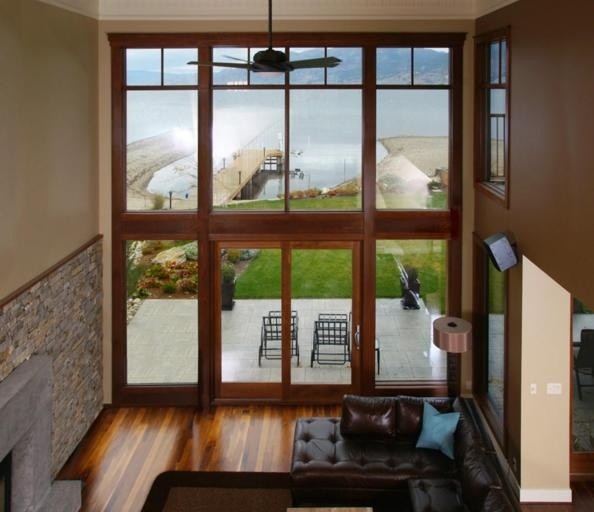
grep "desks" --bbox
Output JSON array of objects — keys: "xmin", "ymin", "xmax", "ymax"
[{"xmin": 286, "ymin": 507, "xmax": 373, "ymax": 512}]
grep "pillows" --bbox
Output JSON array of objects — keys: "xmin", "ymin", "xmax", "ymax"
[{"xmin": 415, "ymin": 401, "xmax": 461, "ymax": 460}]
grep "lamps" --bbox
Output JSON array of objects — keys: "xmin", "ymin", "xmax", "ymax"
[{"xmin": 433, "ymin": 317, "xmax": 473, "ymax": 396}]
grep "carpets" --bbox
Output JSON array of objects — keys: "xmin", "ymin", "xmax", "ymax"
[{"xmin": 140, "ymin": 471, "xmax": 293, "ymax": 512}]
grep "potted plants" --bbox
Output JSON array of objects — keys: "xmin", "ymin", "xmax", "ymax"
[{"xmin": 222, "ymin": 262, "xmax": 235, "ymax": 310}]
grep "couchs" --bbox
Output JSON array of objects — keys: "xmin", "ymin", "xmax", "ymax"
[{"xmin": 289, "ymin": 393, "xmax": 520, "ymax": 512}]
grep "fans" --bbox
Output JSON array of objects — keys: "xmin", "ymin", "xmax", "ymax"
[{"xmin": 187, "ymin": 0, "xmax": 342, "ymax": 72}]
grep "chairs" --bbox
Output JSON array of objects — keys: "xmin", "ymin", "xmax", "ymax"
[
  {"xmin": 310, "ymin": 314, "xmax": 350, "ymax": 368},
  {"xmin": 574, "ymin": 329, "xmax": 594, "ymax": 400},
  {"xmin": 258, "ymin": 310, "xmax": 299, "ymax": 367}
]
